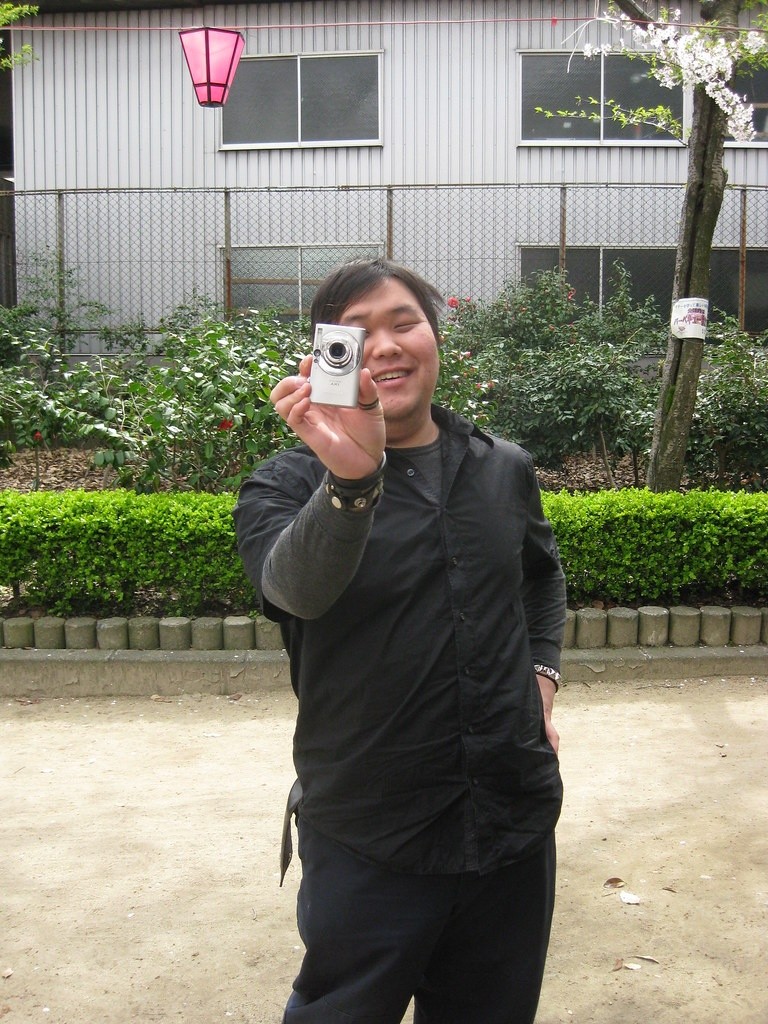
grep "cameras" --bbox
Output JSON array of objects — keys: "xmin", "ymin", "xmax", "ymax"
[{"xmin": 308, "ymin": 321, "xmax": 368, "ymax": 406}]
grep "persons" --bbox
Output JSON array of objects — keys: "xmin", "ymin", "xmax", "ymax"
[{"xmin": 227, "ymin": 257, "xmax": 570, "ymax": 1024}]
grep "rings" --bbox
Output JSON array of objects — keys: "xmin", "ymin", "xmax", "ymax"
[{"xmin": 358, "ymin": 395, "xmax": 380, "ymax": 410}]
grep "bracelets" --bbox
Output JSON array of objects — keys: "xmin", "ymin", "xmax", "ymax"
[
  {"xmin": 322, "ymin": 469, "xmax": 385, "ymax": 511},
  {"xmin": 533, "ymin": 664, "xmax": 562, "ymax": 693}
]
